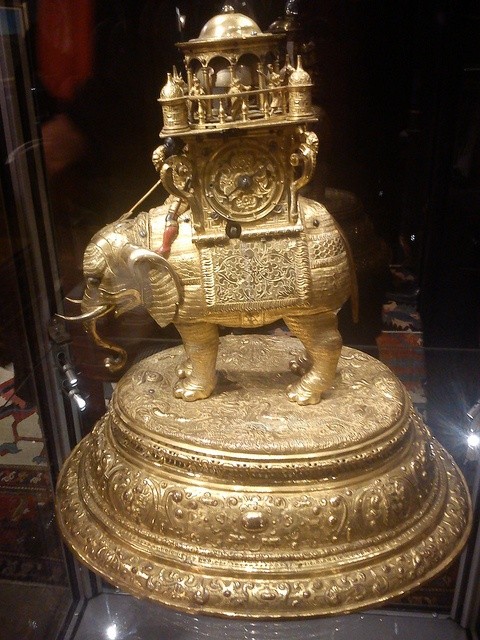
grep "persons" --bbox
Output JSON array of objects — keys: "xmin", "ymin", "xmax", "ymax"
[{"xmin": 188, "ymin": 64, "xmax": 292, "ymax": 123}]
